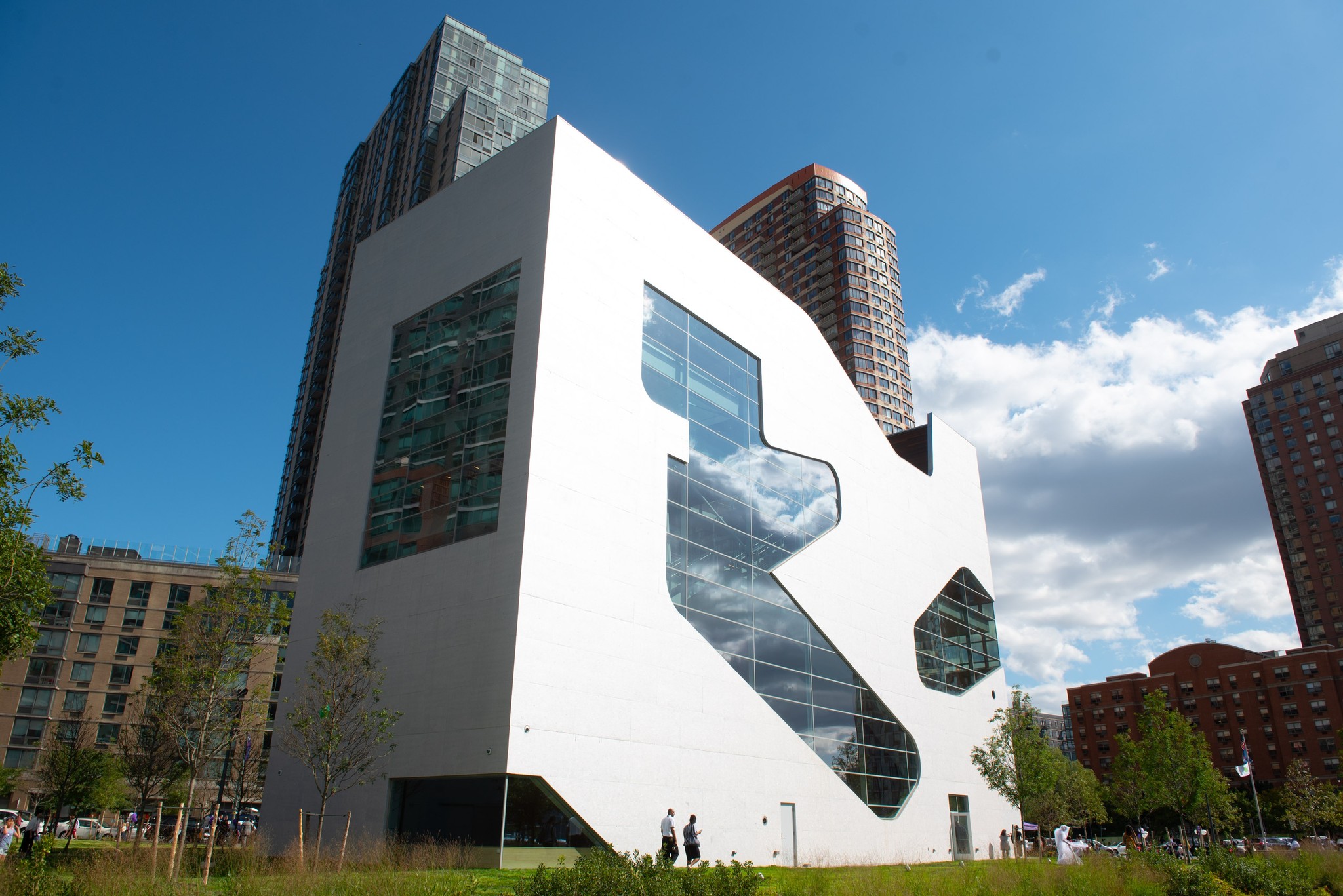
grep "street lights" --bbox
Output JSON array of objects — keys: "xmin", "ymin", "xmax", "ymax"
[{"xmin": 217, "ymin": 688, "xmax": 248, "ymax": 812}]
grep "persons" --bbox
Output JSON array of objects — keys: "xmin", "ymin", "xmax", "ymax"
[
  {"xmin": 1291, "ymin": 835, "xmax": 1337, "ymax": 851},
  {"xmin": 1230, "ymin": 836, "xmax": 1254, "ymax": 858},
  {"xmin": 121, "ymin": 820, "xmax": 129, "ymax": 842},
  {"xmin": 1010, "ymin": 825, "xmax": 1023, "ymax": 858},
  {"xmin": 213, "ymin": 814, "xmax": 256, "ymax": 848},
  {"xmin": 536, "ymin": 816, "xmax": 559, "ymax": 847},
  {"xmin": 1160, "ymin": 834, "xmax": 1194, "ymax": 860},
  {"xmin": 61, "ymin": 814, "xmax": 79, "ymax": 840},
  {"xmin": 1122, "ymin": 825, "xmax": 1136, "ymax": 861},
  {"xmin": 999, "ymin": 829, "xmax": 1010, "ymax": 859},
  {"xmin": 952, "ymin": 821, "xmax": 968, "ymax": 854},
  {"xmin": 1033, "ymin": 835, "xmax": 1046, "ymax": 850},
  {"xmin": 683, "ymin": 815, "xmax": 703, "ymax": 869},
  {"xmin": 1025, "ymin": 836, "xmax": 1028, "ymax": 850},
  {"xmin": 0, "ymin": 810, "xmax": 45, "ymax": 863},
  {"xmin": 566, "ymin": 816, "xmax": 584, "ymax": 848},
  {"xmin": 1054, "ymin": 825, "xmax": 1100, "ymax": 866},
  {"xmin": 661, "ymin": 809, "xmax": 679, "ymax": 868}
]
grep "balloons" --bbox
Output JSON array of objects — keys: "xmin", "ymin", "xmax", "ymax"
[
  {"xmin": 127, "ymin": 812, "xmax": 138, "ymax": 822},
  {"xmin": 1138, "ymin": 827, "xmax": 1148, "ymax": 839},
  {"xmin": 1194, "ymin": 829, "xmax": 1197, "ymax": 834},
  {"xmin": 1201, "ymin": 829, "xmax": 1207, "ymax": 835},
  {"xmin": 203, "ymin": 816, "xmax": 210, "ymax": 825}
]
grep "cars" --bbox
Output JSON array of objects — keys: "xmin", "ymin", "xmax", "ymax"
[
  {"xmin": 0, "ymin": 808, "xmax": 45, "ymax": 837},
  {"xmin": 1028, "ymin": 831, "xmax": 1343, "ymax": 857},
  {"xmin": 52, "ymin": 817, "xmax": 120, "ymax": 841},
  {"xmin": 126, "ymin": 822, "xmax": 150, "ymax": 840}
]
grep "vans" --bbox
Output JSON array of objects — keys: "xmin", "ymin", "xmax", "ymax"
[
  {"xmin": 143, "ymin": 814, "xmax": 204, "ymax": 844},
  {"xmin": 195, "ymin": 813, "xmax": 257, "ymax": 847}
]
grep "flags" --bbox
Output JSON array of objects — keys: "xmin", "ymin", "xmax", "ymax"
[
  {"xmin": 1240, "ymin": 732, "xmax": 1253, "ymax": 764},
  {"xmin": 1235, "ymin": 761, "xmax": 1250, "ymax": 777}
]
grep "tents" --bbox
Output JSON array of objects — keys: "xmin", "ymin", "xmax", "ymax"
[{"xmin": 1023, "ymin": 822, "xmax": 1039, "ymax": 831}]
